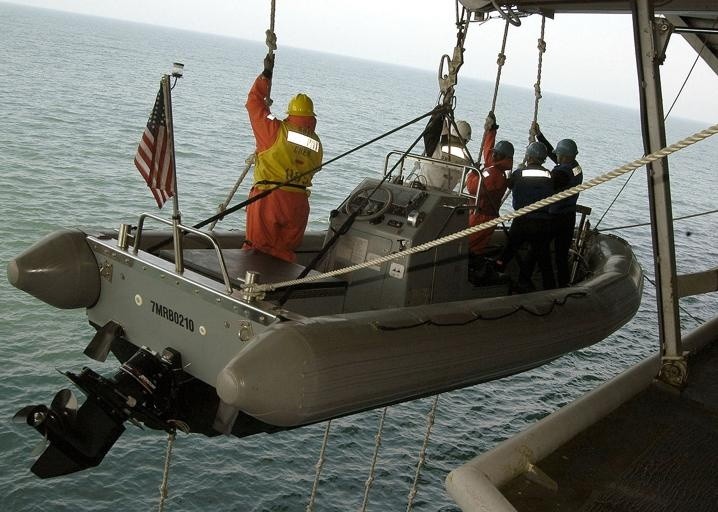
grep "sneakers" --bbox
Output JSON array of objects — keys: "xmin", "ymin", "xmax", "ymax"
[
  {"xmin": 520, "ymin": 279, "xmax": 535, "ymax": 290},
  {"xmin": 486, "ymin": 256, "xmax": 504, "ymax": 273}
]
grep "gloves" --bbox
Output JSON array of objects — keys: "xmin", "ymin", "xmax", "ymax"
[
  {"xmin": 529, "ymin": 122, "xmax": 540, "ymax": 142},
  {"xmin": 473, "ymin": 163, "xmax": 481, "ymax": 174},
  {"xmin": 488, "ymin": 111, "xmax": 499, "ymax": 129}
]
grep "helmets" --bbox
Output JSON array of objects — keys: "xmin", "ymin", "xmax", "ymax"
[
  {"xmin": 526, "ymin": 142, "xmax": 547, "ymax": 160},
  {"xmin": 552, "ymin": 139, "xmax": 578, "ymax": 156},
  {"xmin": 452, "ymin": 121, "xmax": 472, "ymax": 141},
  {"xmin": 492, "ymin": 141, "xmax": 514, "ymax": 156},
  {"xmin": 286, "ymin": 94, "xmax": 317, "ymax": 116}
]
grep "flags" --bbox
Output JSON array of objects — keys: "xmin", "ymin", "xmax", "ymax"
[{"xmin": 133, "ymin": 76, "xmax": 175, "ymax": 209}]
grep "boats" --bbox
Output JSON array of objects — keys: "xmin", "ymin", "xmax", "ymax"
[{"xmin": 7, "ymin": 148, "xmax": 642, "ymax": 480}]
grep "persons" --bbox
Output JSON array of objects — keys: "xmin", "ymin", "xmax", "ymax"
[
  {"xmin": 242, "ymin": 55, "xmax": 323, "ymax": 262},
  {"xmin": 418, "ymin": 88, "xmax": 472, "ymax": 191},
  {"xmin": 494, "ymin": 141, "xmax": 556, "ymax": 290},
  {"xmin": 534, "ymin": 123, "xmax": 583, "ymax": 289},
  {"xmin": 465, "ymin": 111, "xmax": 513, "ymax": 255}
]
